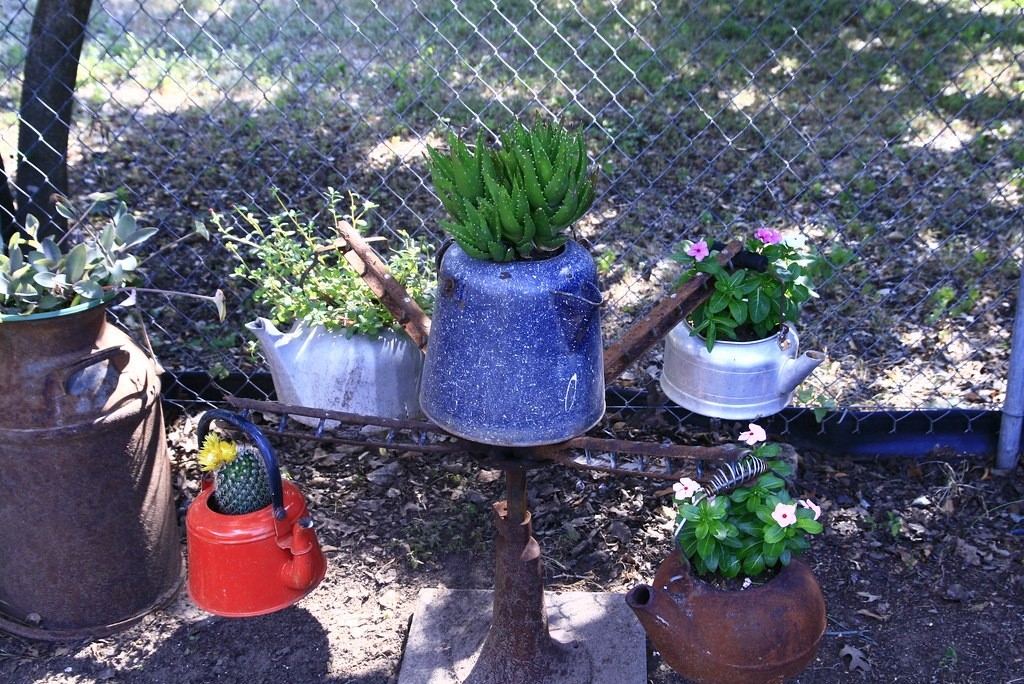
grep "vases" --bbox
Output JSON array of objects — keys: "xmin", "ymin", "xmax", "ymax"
[
  {"xmin": 187, "ymin": 477, "xmax": 327, "ymax": 616},
  {"xmin": 625, "ymin": 549, "xmax": 827, "ymax": 684},
  {"xmin": 658, "ymin": 317, "xmax": 826, "ymax": 423}
]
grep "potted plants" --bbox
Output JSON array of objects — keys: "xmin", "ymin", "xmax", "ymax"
[
  {"xmin": 0, "ymin": 189, "xmax": 228, "ymax": 643},
  {"xmin": 418, "ymin": 108, "xmax": 608, "ymax": 449},
  {"xmin": 207, "ymin": 185, "xmax": 437, "ymax": 435}
]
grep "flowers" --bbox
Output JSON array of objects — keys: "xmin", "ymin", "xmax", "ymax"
[
  {"xmin": 668, "ymin": 229, "xmax": 819, "ymax": 353},
  {"xmin": 196, "ymin": 432, "xmax": 273, "ymax": 516},
  {"xmin": 671, "ymin": 424, "xmax": 825, "ymax": 591}
]
